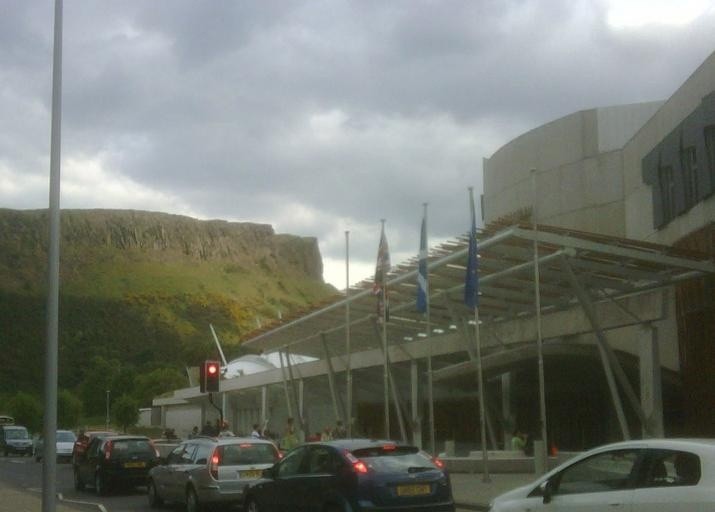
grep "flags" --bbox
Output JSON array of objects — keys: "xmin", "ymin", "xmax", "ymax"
[
  {"xmin": 416, "ymin": 218, "xmax": 426, "ymax": 310},
  {"xmin": 465, "ymin": 200, "xmax": 480, "ymax": 307},
  {"xmin": 373, "ymin": 229, "xmax": 392, "ymax": 324}
]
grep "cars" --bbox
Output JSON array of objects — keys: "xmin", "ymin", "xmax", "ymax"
[
  {"xmin": 1, "ymin": 426, "xmax": 33, "ymax": 456},
  {"xmin": 490, "ymin": 437, "xmax": 715, "ymax": 512}
]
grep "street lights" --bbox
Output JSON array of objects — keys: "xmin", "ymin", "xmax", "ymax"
[{"xmin": 105, "ymin": 390, "xmax": 111, "ymax": 431}]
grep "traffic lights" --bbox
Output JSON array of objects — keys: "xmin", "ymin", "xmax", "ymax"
[{"xmin": 205, "ymin": 361, "xmax": 220, "ymax": 393}]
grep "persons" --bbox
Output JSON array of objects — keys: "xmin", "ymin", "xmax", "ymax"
[
  {"xmin": 511, "ymin": 430, "xmax": 527, "ymax": 451},
  {"xmin": 156, "ymin": 418, "xmax": 347, "ymax": 453}
]
